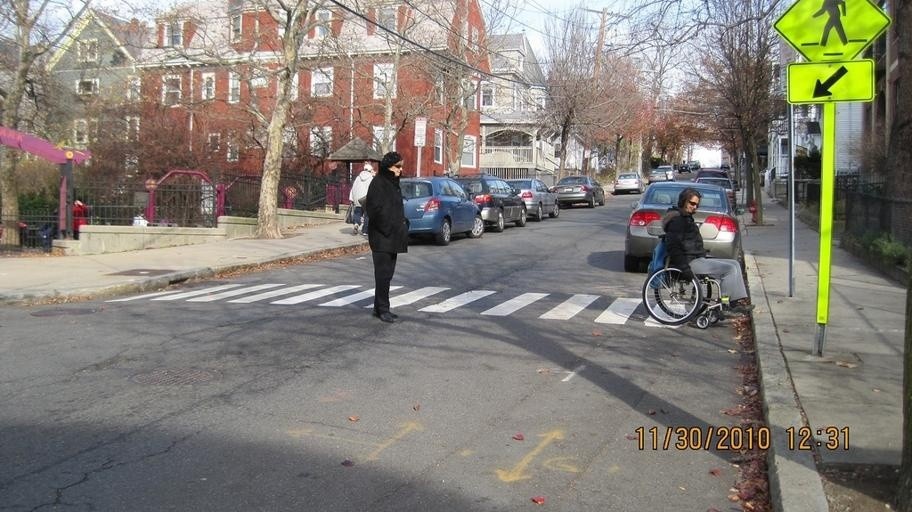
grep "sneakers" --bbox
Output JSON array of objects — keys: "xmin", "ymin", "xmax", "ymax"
[
  {"xmin": 362, "ymin": 232, "xmax": 368, "ymax": 236},
  {"xmin": 728, "ymin": 299, "xmax": 757, "ymax": 313},
  {"xmin": 353, "ymin": 225, "xmax": 359, "ymax": 235}
]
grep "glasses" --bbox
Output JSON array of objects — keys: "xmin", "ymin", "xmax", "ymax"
[
  {"xmin": 391, "ymin": 164, "xmax": 404, "ymax": 169},
  {"xmin": 688, "ymin": 200, "xmax": 700, "ymax": 209}
]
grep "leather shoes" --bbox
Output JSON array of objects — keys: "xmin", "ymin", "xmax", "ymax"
[{"xmin": 372, "ymin": 308, "xmax": 399, "ymax": 323}]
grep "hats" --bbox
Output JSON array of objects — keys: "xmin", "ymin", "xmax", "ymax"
[
  {"xmin": 380, "ymin": 151, "xmax": 402, "ymax": 168},
  {"xmin": 363, "ymin": 160, "xmax": 374, "ymax": 171}
]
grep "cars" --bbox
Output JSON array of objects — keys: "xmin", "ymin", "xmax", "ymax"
[
  {"xmin": 553, "ymin": 175, "xmax": 605, "ymax": 209},
  {"xmin": 398, "ymin": 177, "xmax": 485, "ymax": 245},
  {"xmin": 506, "ymin": 179, "xmax": 561, "ymax": 222},
  {"xmin": 612, "ymin": 159, "xmax": 741, "ymax": 272}
]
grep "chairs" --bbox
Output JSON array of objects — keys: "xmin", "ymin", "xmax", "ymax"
[
  {"xmin": 700, "ymin": 198, "xmax": 713, "ymax": 206},
  {"xmin": 656, "ymin": 194, "xmax": 672, "ymax": 204}
]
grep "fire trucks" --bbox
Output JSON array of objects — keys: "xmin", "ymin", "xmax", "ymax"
[{"xmin": 749, "ymin": 199, "xmax": 757, "ymax": 222}]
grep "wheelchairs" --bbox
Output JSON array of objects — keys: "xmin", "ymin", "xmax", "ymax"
[{"xmin": 643, "ymin": 233, "xmax": 731, "ymax": 330}]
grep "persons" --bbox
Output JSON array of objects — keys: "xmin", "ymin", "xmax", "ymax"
[
  {"xmin": 72, "ymin": 196, "xmax": 88, "ymax": 239},
  {"xmin": 365, "ymin": 150, "xmax": 410, "ymax": 323},
  {"xmin": 348, "ymin": 164, "xmax": 375, "ymax": 236},
  {"xmin": 663, "ymin": 186, "xmax": 756, "ymax": 313}
]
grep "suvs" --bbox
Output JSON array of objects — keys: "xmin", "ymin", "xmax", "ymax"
[{"xmin": 449, "ymin": 174, "xmax": 527, "ymax": 232}]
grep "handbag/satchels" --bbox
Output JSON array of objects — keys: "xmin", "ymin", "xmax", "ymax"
[{"xmin": 344, "ymin": 202, "xmax": 355, "ymax": 224}]
